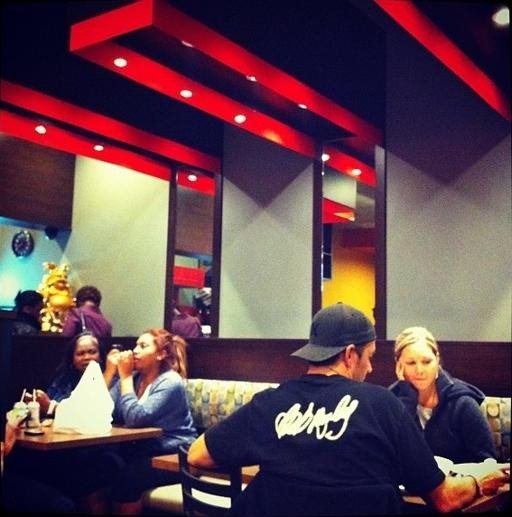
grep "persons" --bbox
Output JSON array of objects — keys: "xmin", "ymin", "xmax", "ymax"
[
  {"xmin": 63, "ymin": 286, "xmax": 112, "ymax": 337},
  {"xmin": 0, "ymin": 290, "xmax": 45, "ymax": 335},
  {"xmin": 171, "ymin": 298, "xmax": 204, "ymax": 337},
  {"xmin": 187, "ymin": 302, "xmax": 510, "ymax": 517},
  {"xmin": 387, "ymin": 327, "xmax": 497, "ymax": 463},
  {"xmin": 0, "ymin": 407, "xmax": 31, "ymax": 473},
  {"xmin": 83, "ymin": 328, "xmax": 200, "ymax": 517},
  {"xmin": 25, "ymin": 332, "xmax": 101, "ymax": 417}
]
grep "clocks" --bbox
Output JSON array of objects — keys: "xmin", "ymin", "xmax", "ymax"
[{"xmin": 13, "ymin": 230, "xmax": 34, "ymax": 257}]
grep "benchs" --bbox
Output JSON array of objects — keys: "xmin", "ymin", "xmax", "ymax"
[{"xmin": 150, "ymin": 373, "xmax": 511, "ymax": 513}]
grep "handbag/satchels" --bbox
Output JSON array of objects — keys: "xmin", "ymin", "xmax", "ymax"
[{"xmin": 52, "ymin": 359, "xmax": 114, "ymax": 435}]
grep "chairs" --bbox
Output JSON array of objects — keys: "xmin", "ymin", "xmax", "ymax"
[{"xmin": 177, "ymin": 445, "xmax": 244, "ymax": 516}]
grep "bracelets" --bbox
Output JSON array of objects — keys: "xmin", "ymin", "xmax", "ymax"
[
  {"xmin": 46, "ymin": 399, "xmax": 57, "ymax": 415},
  {"xmin": 467, "ymin": 475, "xmax": 483, "ymax": 498}
]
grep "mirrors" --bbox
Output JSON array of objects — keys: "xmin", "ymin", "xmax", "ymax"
[
  {"xmin": 165, "ymin": 162, "xmax": 223, "ymax": 340},
  {"xmin": 312, "ymin": 136, "xmax": 387, "ymax": 340}
]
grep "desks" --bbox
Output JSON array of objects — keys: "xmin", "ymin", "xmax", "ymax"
[
  {"xmin": 151, "ymin": 450, "xmax": 512, "ymax": 514},
  {"xmin": 16, "ymin": 427, "xmax": 163, "ymax": 452}
]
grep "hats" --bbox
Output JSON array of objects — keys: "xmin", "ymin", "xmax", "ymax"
[{"xmin": 290, "ymin": 301, "xmax": 376, "ymax": 362}]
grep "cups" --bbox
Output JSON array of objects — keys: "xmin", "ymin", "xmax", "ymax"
[
  {"xmin": 28, "ymin": 401, "xmax": 40, "ymax": 429},
  {"xmin": 13, "ymin": 402, "xmax": 26, "ymax": 430}
]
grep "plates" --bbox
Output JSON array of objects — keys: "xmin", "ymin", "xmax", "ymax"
[{"xmin": 449, "ymin": 461, "xmax": 505, "ymax": 479}]
